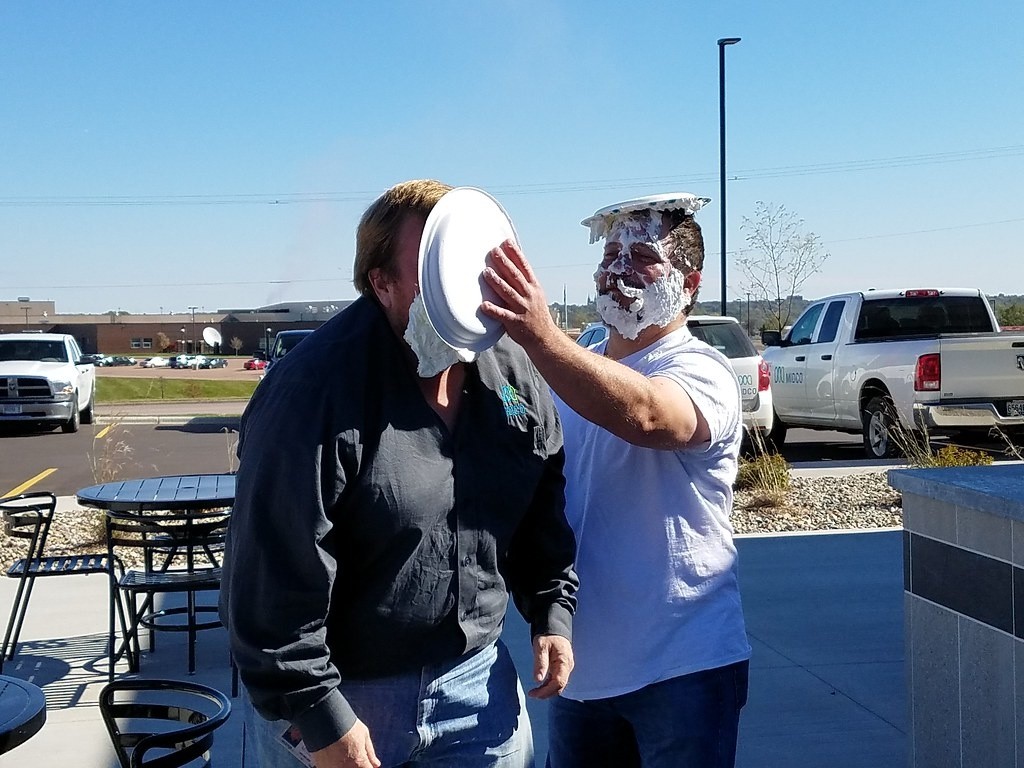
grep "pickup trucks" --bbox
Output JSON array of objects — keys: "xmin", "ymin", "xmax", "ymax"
[{"xmin": 761, "ymin": 288, "xmax": 1023, "ymax": 462}]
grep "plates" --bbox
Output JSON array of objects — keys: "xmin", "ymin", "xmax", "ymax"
[
  {"xmin": 417, "ymin": 186, "xmax": 522, "ymax": 354},
  {"xmin": 579, "ymin": 191, "xmax": 714, "ymax": 232}
]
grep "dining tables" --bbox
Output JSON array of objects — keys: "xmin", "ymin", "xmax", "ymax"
[
  {"xmin": 0, "ymin": 674, "xmax": 47, "ymax": 756},
  {"xmin": 75, "ymin": 474, "xmax": 250, "ymax": 675}
]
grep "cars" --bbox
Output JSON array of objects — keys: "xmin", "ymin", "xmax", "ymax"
[
  {"xmin": 575, "ymin": 315, "xmax": 773, "ymax": 463},
  {"xmin": 243, "ymin": 359, "xmax": 268, "ymax": 370},
  {"xmin": 265, "ymin": 329, "xmax": 317, "ymax": 375},
  {"xmin": 86, "ymin": 353, "xmax": 229, "ymax": 370},
  {"xmin": 0, "ymin": 334, "xmax": 97, "ymax": 433}
]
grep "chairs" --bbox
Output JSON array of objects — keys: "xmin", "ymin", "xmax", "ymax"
[
  {"xmin": 104, "ymin": 508, "xmax": 240, "ymax": 699},
  {"xmin": 99, "ymin": 678, "xmax": 232, "ymax": 768},
  {"xmin": 0, "ymin": 491, "xmax": 141, "ymax": 676}
]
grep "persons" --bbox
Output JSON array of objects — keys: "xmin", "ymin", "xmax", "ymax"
[
  {"xmin": 218, "ymin": 180, "xmax": 581, "ymax": 768},
  {"xmin": 478, "ymin": 205, "xmax": 751, "ymax": 767}
]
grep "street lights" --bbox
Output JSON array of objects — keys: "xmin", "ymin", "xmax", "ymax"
[
  {"xmin": 188, "ymin": 307, "xmax": 198, "ymax": 353},
  {"xmin": 266, "ymin": 327, "xmax": 271, "ymax": 360},
  {"xmin": 20, "ymin": 307, "xmax": 32, "ymax": 330},
  {"xmin": 718, "ymin": 37, "xmax": 742, "ymax": 317}
]
